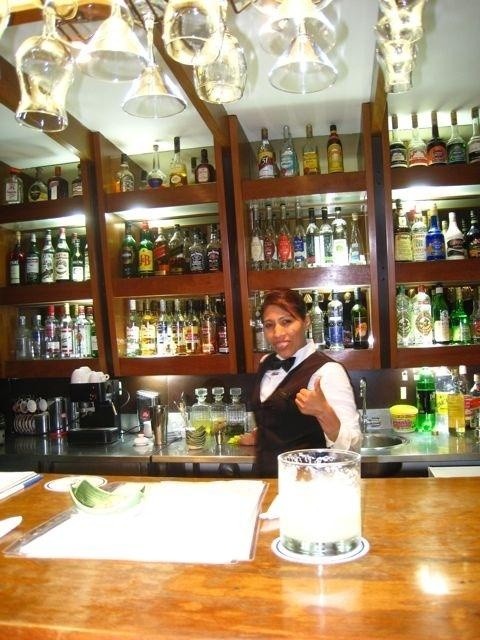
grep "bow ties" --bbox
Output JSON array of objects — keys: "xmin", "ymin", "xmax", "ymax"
[{"xmin": 264, "ymin": 355, "xmax": 296, "ymax": 373}]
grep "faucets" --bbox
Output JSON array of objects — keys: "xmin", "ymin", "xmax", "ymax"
[{"xmin": 358, "ymin": 377, "xmax": 368, "ymax": 420}]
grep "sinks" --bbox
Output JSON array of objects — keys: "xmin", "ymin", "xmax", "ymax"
[{"xmin": 360, "ymin": 433, "xmax": 403, "ymax": 450}]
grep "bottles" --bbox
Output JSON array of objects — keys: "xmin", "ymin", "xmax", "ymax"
[
  {"xmin": 280, "ymin": 125, "xmax": 299, "ymax": 177},
  {"xmin": 326, "ymin": 125, "xmax": 344, "ymax": 174},
  {"xmin": 254, "ymin": 287, "xmax": 477, "ymax": 353},
  {"xmin": 188, "ymin": 381, "xmax": 248, "ymax": 440},
  {"xmin": 388, "ymin": 108, "xmax": 480, "ymax": 169},
  {"xmin": 257, "ymin": 128, "xmax": 280, "ymax": 179},
  {"xmin": 124, "ymin": 292, "xmax": 228, "ymax": 361},
  {"xmin": 413, "ymin": 357, "xmax": 480, "ymax": 440},
  {"xmin": 189, "ymin": 148, "xmax": 215, "ymax": 184},
  {"xmin": 11, "ymin": 304, "xmax": 97, "ymax": 362},
  {"xmin": 116, "ymin": 152, "xmax": 134, "ymax": 192},
  {"xmin": 2, "ymin": 159, "xmax": 82, "ymax": 206},
  {"xmin": 141, "ymin": 145, "xmax": 167, "ymax": 189},
  {"xmin": 169, "ymin": 137, "xmax": 187, "ymax": 186},
  {"xmin": 245, "ymin": 202, "xmax": 478, "ymax": 273},
  {"xmin": 301, "ymin": 125, "xmax": 321, "ymax": 176},
  {"xmin": 9, "ymin": 225, "xmax": 90, "ymax": 285},
  {"xmin": 121, "ymin": 217, "xmax": 219, "ymax": 278}
]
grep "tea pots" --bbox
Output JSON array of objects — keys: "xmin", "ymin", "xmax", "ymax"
[{"xmin": 148, "ymin": 405, "xmax": 168, "ymax": 446}]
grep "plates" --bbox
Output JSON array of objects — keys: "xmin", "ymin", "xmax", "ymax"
[
  {"xmin": 14, "ymin": 416, "xmax": 35, "ymax": 433},
  {"xmin": 165, "ymin": 431, "xmax": 181, "ymax": 441}
]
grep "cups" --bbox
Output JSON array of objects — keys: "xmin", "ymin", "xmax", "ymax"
[
  {"xmin": 72, "ymin": 366, "xmax": 108, "ymax": 382},
  {"xmin": 136, "ymin": 390, "xmax": 162, "ymax": 434},
  {"xmin": 12, "ymin": 396, "xmax": 46, "ymax": 415},
  {"xmin": 12, "ymin": 1, "xmax": 424, "ymax": 137},
  {"xmin": 142, "ymin": 421, "xmax": 152, "ymax": 438}
]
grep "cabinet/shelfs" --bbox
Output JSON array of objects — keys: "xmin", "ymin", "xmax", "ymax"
[
  {"xmin": 105, "ymin": 181, "xmax": 238, "ymax": 373},
  {"xmin": 387, "ymin": 163, "xmax": 478, "ymax": 366},
  {"xmin": 240, "ymin": 169, "xmax": 383, "ymax": 369},
  {"xmin": 0, "ymin": 458, "xmax": 157, "ymax": 477},
  {"xmin": 2, "ymin": 196, "xmax": 106, "ymax": 374}
]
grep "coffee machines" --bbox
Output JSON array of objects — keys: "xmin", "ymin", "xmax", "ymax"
[{"xmin": 68, "ymin": 379, "xmax": 124, "ymax": 446}]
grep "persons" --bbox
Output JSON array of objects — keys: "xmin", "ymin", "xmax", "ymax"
[{"xmin": 238, "ymin": 289, "xmax": 361, "ymax": 479}]
